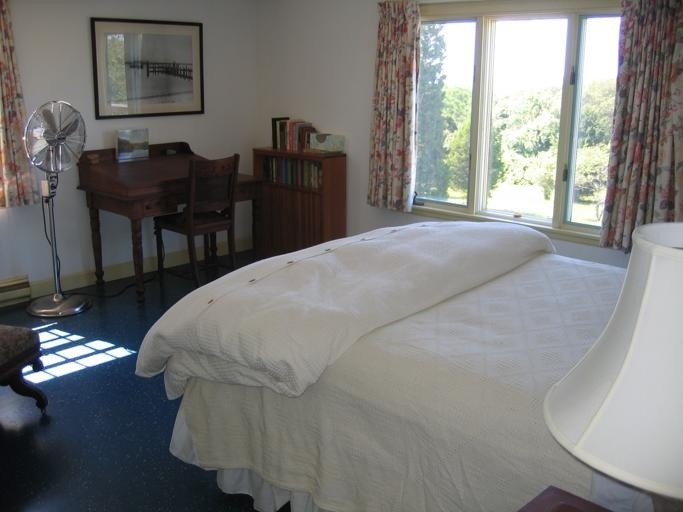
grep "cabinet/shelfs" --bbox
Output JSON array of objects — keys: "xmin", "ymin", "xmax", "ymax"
[{"xmin": 251, "ymin": 147, "xmax": 349, "ymax": 259}]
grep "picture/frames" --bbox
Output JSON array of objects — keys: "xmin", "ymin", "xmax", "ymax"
[{"xmin": 89, "ymin": 15, "xmax": 206, "ymax": 120}]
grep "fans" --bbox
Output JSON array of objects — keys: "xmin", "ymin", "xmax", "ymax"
[{"xmin": 21, "ymin": 99, "xmax": 95, "ymax": 319}]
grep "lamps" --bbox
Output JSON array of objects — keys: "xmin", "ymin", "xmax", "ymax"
[{"xmin": 544, "ymin": 221, "xmax": 683, "ymax": 511}]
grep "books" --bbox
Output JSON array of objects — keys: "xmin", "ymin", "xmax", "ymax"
[
  {"xmin": 272, "ymin": 157, "xmax": 320, "ymax": 188},
  {"xmin": 272, "ymin": 116, "xmax": 316, "ymax": 152}
]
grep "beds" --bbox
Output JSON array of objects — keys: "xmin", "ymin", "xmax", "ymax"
[{"xmin": 172, "ymin": 219, "xmax": 653, "ymax": 512}]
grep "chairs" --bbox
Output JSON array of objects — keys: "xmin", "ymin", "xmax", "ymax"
[{"xmin": 153, "ymin": 152, "xmax": 241, "ymax": 309}]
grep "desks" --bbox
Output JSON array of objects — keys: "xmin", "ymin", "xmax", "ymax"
[{"xmin": 74, "ymin": 144, "xmax": 257, "ymax": 304}]
